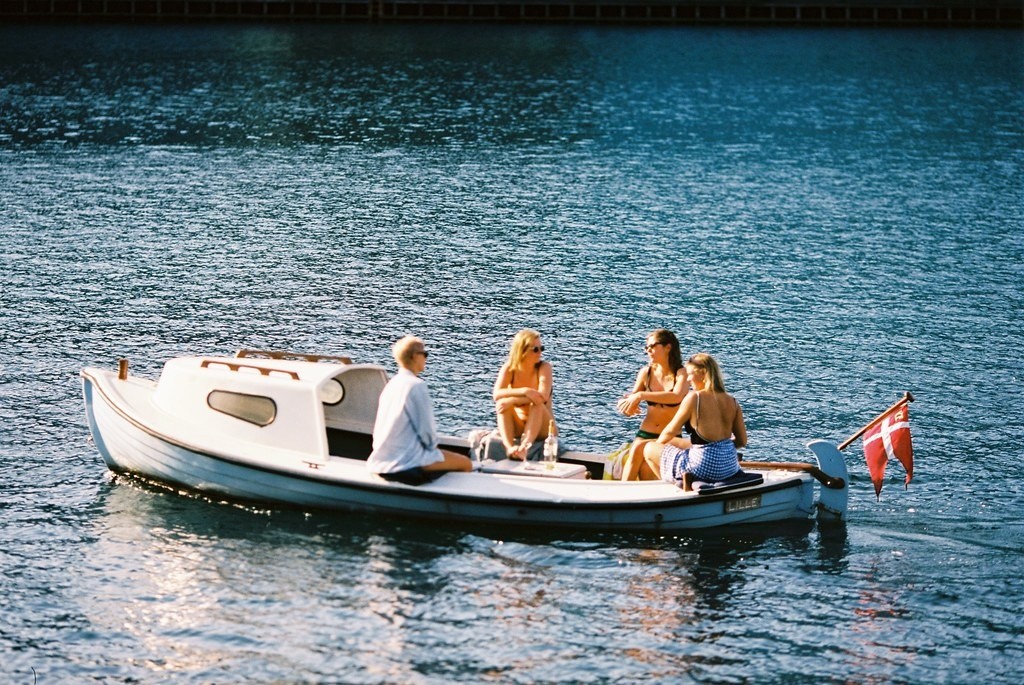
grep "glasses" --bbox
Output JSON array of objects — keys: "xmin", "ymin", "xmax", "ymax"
[
  {"xmin": 688, "ymin": 357, "xmax": 702, "ymax": 368},
  {"xmin": 527, "ymin": 345, "xmax": 545, "ymax": 353},
  {"xmin": 644, "ymin": 342, "xmax": 663, "ymax": 350},
  {"xmin": 417, "ymin": 351, "xmax": 429, "ymax": 358}
]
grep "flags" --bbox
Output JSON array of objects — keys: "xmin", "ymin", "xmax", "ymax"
[{"xmin": 864, "ymin": 403, "xmax": 914, "ymax": 502}]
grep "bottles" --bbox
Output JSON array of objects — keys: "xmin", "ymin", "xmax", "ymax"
[{"xmin": 543, "ymin": 420, "xmax": 558, "ymax": 471}]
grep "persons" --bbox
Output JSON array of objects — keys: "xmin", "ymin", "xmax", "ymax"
[
  {"xmin": 367, "ymin": 334, "xmax": 473, "ymax": 485},
  {"xmin": 617, "ymin": 328, "xmax": 689, "ymax": 482},
  {"xmin": 492, "ymin": 328, "xmax": 557, "ymax": 459},
  {"xmin": 643, "ymin": 355, "xmax": 747, "ymax": 482}
]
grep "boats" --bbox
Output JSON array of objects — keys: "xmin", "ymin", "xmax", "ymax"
[{"xmin": 81, "ymin": 345, "xmax": 850, "ymax": 532}]
